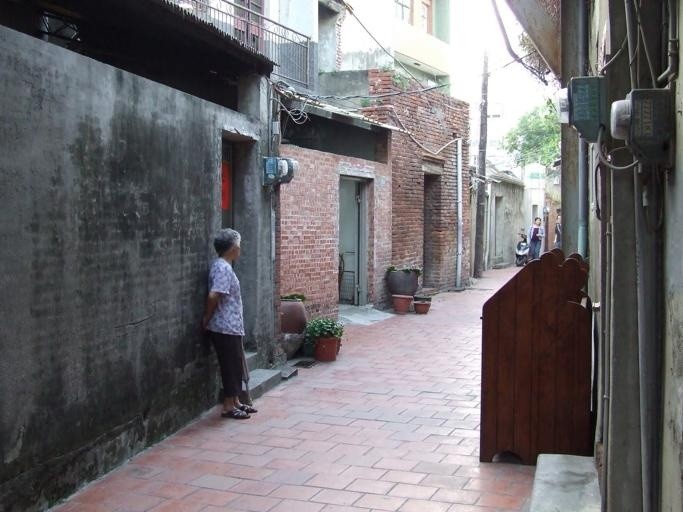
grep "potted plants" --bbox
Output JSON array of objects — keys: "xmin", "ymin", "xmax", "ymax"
[
  {"xmin": 279, "ymin": 294, "xmax": 344, "ymax": 362},
  {"xmin": 386, "ymin": 265, "xmax": 423, "ymax": 296}
]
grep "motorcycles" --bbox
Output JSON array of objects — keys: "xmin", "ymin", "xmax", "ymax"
[{"xmin": 515, "ymin": 226, "xmax": 530, "ymax": 267}]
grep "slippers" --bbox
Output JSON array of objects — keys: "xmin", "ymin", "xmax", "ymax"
[{"xmin": 221, "ymin": 404, "xmax": 257, "ymax": 418}]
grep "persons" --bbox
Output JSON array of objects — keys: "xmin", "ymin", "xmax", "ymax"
[
  {"xmin": 554, "ymin": 215, "xmax": 562, "ymax": 247},
  {"xmin": 526, "ymin": 217, "xmax": 545, "ymax": 261},
  {"xmin": 202, "ymin": 228, "xmax": 258, "ymax": 419}
]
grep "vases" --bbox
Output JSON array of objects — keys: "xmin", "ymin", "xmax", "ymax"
[{"xmin": 391, "ymin": 294, "xmax": 431, "ymax": 314}]
